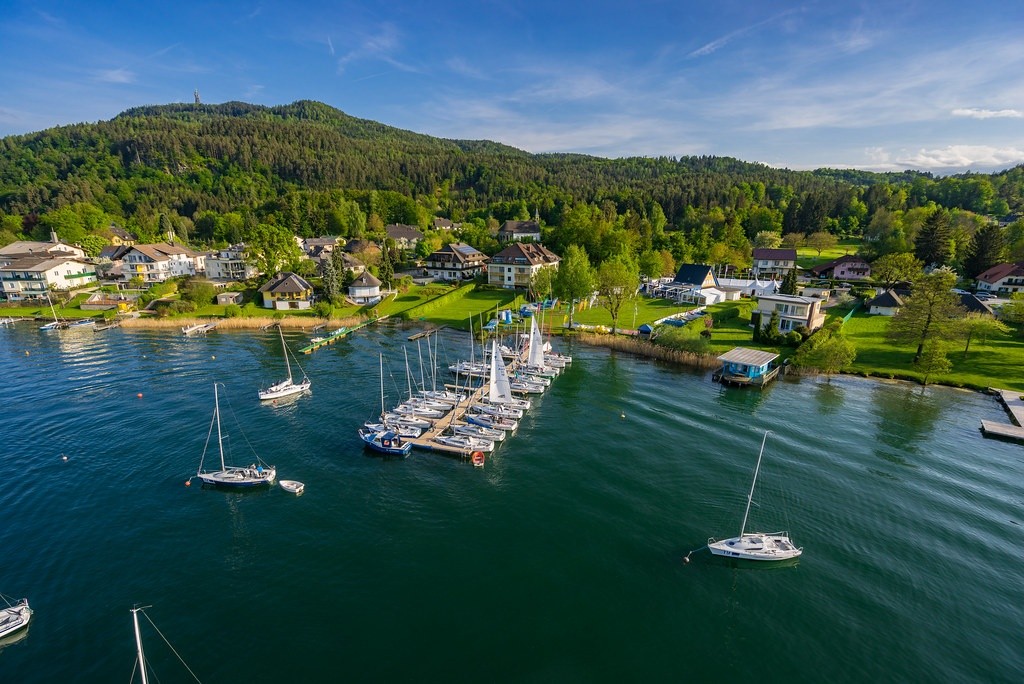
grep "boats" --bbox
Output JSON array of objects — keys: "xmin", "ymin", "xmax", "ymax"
[
  {"xmin": 68, "ymin": 318, "xmax": 96, "ymax": 329},
  {"xmin": 279, "ymin": 479, "xmax": 305, "ymax": 494},
  {"xmin": 470, "ymin": 450, "xmax": 485, "ymax": 467},
  {"xmin": 358, "ymin": 418, "xmax": 414, "ymax": 457},
  {"xmin": 311, "ymin": 336, "xmax": 324, "ymax": 345},
  {"xmin": 329, "ymin": 326, "xmax": 347, "ymax": 337}
]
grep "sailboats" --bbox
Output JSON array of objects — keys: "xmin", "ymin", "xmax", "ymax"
[
  {"xmin": 254, "ymin": 324, "xmax": 311, "ymax": 401},
  {"xmin": 195, "ymin": 380, "xmax": 277, "ymax": 489},
  {"xmin": 0, "ymin": 592, "xmax": 35, "ymax": 641},
  {"xmin": 363, "ymin": 297, "xmax": 574, "ymax": 452},
  {"xmin": 38, "ymin": 295, "xmax": 72, "ymax": 332},
  {"xmin": 706, "ymin": 428, "xmax": 805, "ymax": 562}
]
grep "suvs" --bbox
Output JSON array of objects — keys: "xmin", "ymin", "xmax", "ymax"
[
  {"xmin": 951, "ymin": 289, "xmax": 971, "ymax": 295},
  {"xmin": 975, "ymin": 292, "xmax": 997, "ymax": 299}
]
